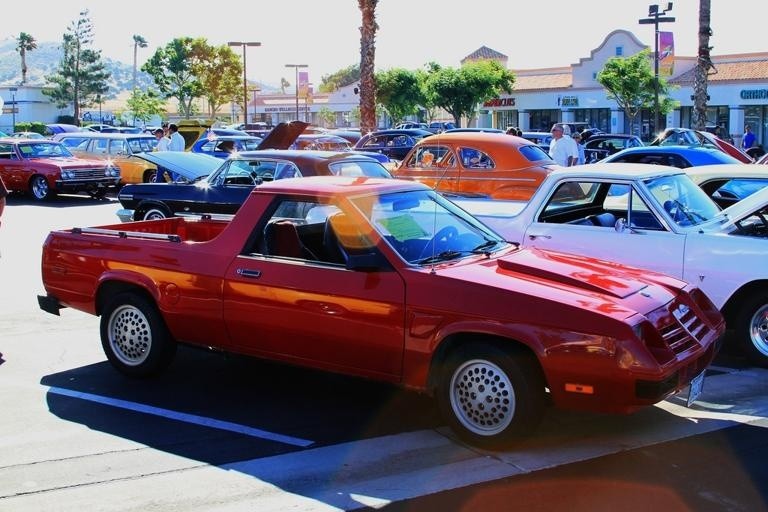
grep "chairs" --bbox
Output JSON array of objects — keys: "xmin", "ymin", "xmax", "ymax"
[
  {"xmin": 264, "ymin": 216, "xmax": 321, "ymax": 272},
  {"xmin": 320, "ymin": 211, "xmax": 373, "ymax": 266}
]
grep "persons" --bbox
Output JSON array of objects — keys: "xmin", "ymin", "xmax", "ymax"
[
  {"xmin": 741, "ymin": 124, "xmax": 757, "ymax": 148},
  {"xmin": 714, "ymin": 126, "xmax": 722, "ymax": 139},
  {"xmin": 154, "ymin": 129, "xmax": 170, "ymax": 183},
  {"xmin": 506, "ymin": 123, "xmax": 586, "ymax": 166},
  {"xmin": 0, "ymin": 177, "xmax": 9, "ymax": 259},
  {"xmin": 167, "ymin": 125, "xmax": 185, "ymax": 181},
  {"xmin": 162, "ymin": 124, "xmax": 169, "ymax": 136}
]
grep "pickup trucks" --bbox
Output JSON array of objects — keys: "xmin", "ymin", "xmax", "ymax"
[
  {"xmin": 303, "ymin": 159, "xmax": 768, "ymax": 369},
  {"xmin": 28, "ymin": 173, "xmax": 728, "ymax": 453}
]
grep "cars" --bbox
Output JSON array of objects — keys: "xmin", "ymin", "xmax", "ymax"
[
  {"xmin": 0, "ymin": 104, "xmax": 767, "ymax": 229},
  {"xmin": 0, "ymin": 138, "xmax": 123, "ymax": 202}
]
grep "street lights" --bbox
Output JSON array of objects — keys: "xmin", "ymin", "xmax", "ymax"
[
  {"xmin": 228, "ymin": 41, "xmax": 261, "ymax": 130},
  {"xmin": 284, "ymin": 64, "xmax": 311, "ymax": 120},
  {"xmin": 639, "ymin": 3, "xmax": 675, "ymax": 140}
]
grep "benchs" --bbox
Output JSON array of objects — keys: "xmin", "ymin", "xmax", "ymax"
[{"xmin": 561, "ymin": 211, "xmax": 616, "ymax": 228}]
